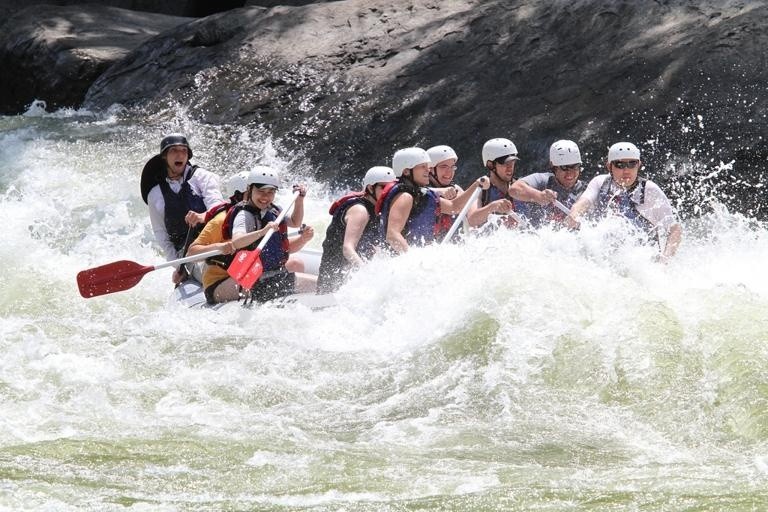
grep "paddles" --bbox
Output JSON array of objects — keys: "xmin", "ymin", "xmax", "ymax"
[
  {"xmin": 227, "ymin": 184, "xmax": 304, "ymax": 289},
  {"xmin": 76, "ymin": 224, "xmax": 307, "ymax": 298}
]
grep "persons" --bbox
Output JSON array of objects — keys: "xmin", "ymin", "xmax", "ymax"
[
  {"xmin": 566, "ymin": 142, "xmax": 682, "ymax": 263},
  {"xmin": 146, "ymin": 133, "xmax": 225, "ymax": 286},
  {"xmin": 376, "ymin": 147, "xmax": 490, "ymax": 256},
  {"xmin": 317, "ymin": 165, "xmax": 396, "ymax": 295},
  {"xmin": 234, "ymin": 167, "xmax": 319, "ymax": 302},
  {"xmin": 427, "ymin": 146, "xmax": 465, "ymax": 244},
  {"xmin": 469, "ymin": 138, "xmax": 521, "ymax": 231},
  {"xmin": 190, "ymin": 172, "xmax": 315, "ymax": 303},
  {"xmin": 510, "ymin": 139, "xmax": 590, "ymax": 230}
]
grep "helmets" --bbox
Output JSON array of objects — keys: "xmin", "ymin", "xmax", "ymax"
[
  {"xmin": 363, "ymin": 165, "xmax": 396, "ymax": 194},
  {"xmin": 426, "ymin": 145, "xmax": 458, "ymax": 168},
  {"xmin": 392, "ymin": 147, "xmax": 432, "ymax": 179},
  {"xmin": 549, "ymin": 139, "xmax": 582, "ymax": 167},
  {"xmin": 247, "ymin": 165, "xmax": 280, "ymax": 191},
  {"xmin": 481, "ymin": 137, "xmax": 518, "ymax": 168},
  {"xmin": 607, "ymin": 141, "xmax": 640, "ymax": 164},
  {"xmin": 159, "ymin": 133, "xmax": 189, "ymax": 156}
]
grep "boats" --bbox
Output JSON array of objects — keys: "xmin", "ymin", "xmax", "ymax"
[{"xmin": 163, "ymin": 227, "xmax": 326, "ymax": 320}]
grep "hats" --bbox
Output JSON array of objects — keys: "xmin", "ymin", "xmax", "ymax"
[
  {"xmin": 253, "ymin": 183, "xmax": 278, "ymax": 191},
  {"xmin": 495, "ymin": 154, "xmax": 520, "ymax": 164}
]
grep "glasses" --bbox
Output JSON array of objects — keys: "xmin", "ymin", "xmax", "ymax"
[
  {"xmin": 376, "ymin": 183, "xmax": 385, "ymax": 190},
  {"xmin": 438, "ymin": 164, "xmax": 457, "ymax": 171},
  {"xmin": 558, "ymin": 163, "xmax": 582, "ymax": 171},
  {"xmin": 611, "ymin": 160, "xmax": 638, "ymax": 169}
]
